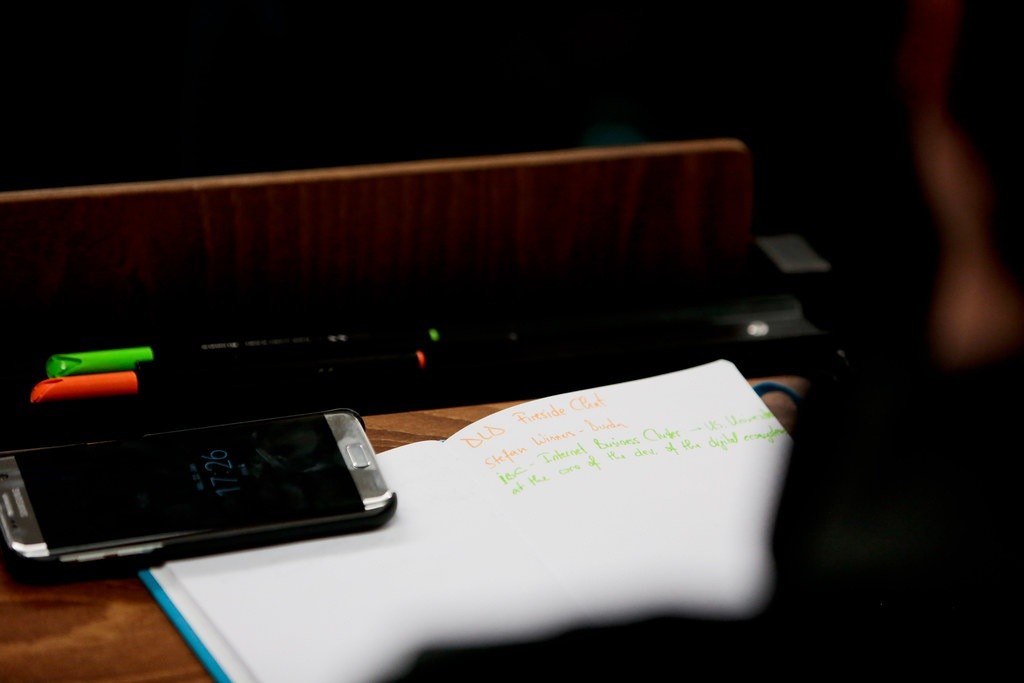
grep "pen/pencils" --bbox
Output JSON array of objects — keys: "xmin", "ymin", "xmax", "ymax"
[{"xmin": 29, "ymin": 326, "xmax": 444, "ymax": 407}]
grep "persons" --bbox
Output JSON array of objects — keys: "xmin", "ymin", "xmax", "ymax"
[{"xmin": 390, "ymin": 0, "xmax": 1023, "ymax": 683}]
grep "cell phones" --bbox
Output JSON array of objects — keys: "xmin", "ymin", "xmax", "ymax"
[{"xmin": 0, "ymin": 409, "xmax": 398, "ymax": 586}]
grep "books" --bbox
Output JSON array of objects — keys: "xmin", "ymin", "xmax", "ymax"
[{"xmin": 138, "ymin": 358, "xmax": 794, "ymax": 682}]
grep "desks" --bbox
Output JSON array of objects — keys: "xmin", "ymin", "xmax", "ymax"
[{"xmin": 0, "ymin": 401, "xmax": 521, "ymax": 682}]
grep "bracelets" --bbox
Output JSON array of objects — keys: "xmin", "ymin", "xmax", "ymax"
[{"xmin": 753, "ymin": 381, "xmax": 802, "ymax": 406}]
